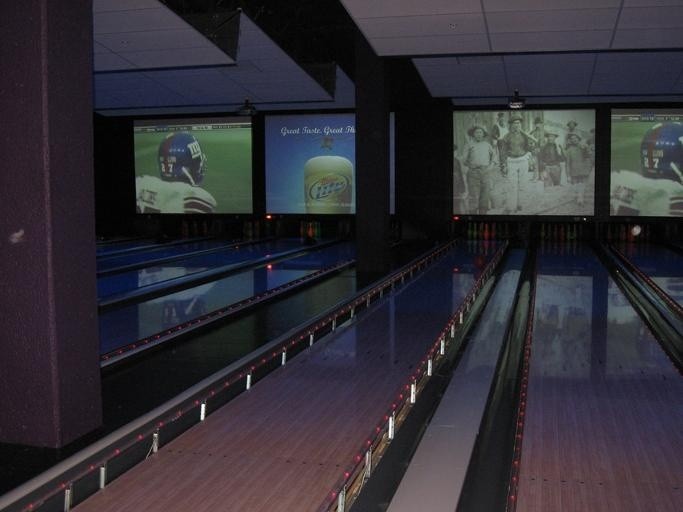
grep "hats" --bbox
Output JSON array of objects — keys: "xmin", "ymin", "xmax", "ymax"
[{"xmin": 467, "ymin": 113, "xmax": 583, "ymax": 141}]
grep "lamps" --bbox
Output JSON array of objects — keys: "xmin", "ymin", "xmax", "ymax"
[
  {"xmin": 236, "ymin": 99, "xmax": 256, "ymax": 115},
  {"xmin": 508, "ymin": 90, "xmax": 524, "ymax": 109}
]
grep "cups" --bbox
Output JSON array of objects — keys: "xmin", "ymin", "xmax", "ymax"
[{"xmin": 303, "ymin": 155, "xmax": 354, "ymax": 214}]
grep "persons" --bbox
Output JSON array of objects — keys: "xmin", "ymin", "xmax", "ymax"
[
  {"xmin": 452, "ymin": 112, "xmax": 594, "ymax": 217},
  {"xmin": 135, "ymin": 131, "xmax": 216, "ymax": 214},
  {"xmin": 609, "ymin": 121, "xmax": 682, "ymax": 216}
]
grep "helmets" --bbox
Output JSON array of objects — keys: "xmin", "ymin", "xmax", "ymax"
[
  {"xmin": 158, "ymin": 131, "xmax": 208, "ymax": 187},
  {"xmin": 640, "ymin": 120, "xmax": 683, "ymax": 181}
]
